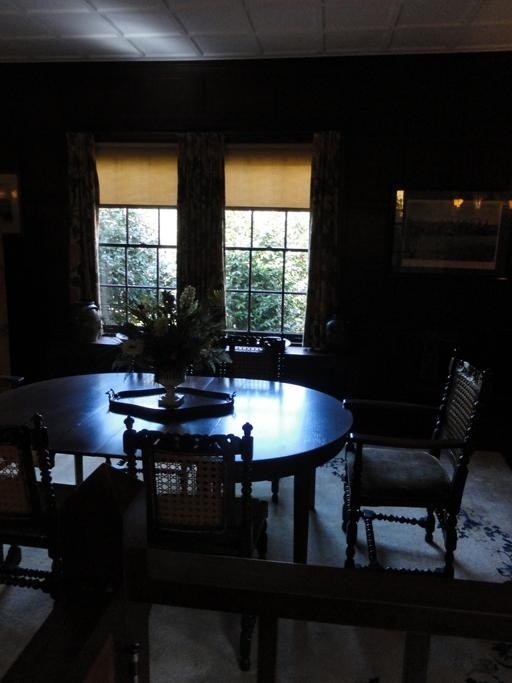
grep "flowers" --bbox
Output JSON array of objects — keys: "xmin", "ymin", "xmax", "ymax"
[{"xmin": 94, "ymin": 282, "xmax": 234, "ymax": 375}]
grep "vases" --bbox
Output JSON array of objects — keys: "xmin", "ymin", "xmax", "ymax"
[{"xmin": 154, "ymin": 367, "xmax": 186, "ymax": 408}]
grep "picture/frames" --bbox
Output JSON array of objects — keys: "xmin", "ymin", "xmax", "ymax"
[
  {"xmin": 379, "ymin": 180, "xmax": 511, "ymax": 290},
  {"xmin": 0, "ymin": 171, "xmax": 23, "ymax": 236}
]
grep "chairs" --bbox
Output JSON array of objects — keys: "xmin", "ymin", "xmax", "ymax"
[
  {"xmin": 339, "ymin": 343, "xmax": 492, "ymax": 567},
  {"xmin": 0, "ymin": 408, "xmax": 143, "ymax": 607},
  {"xmin": 122, "ymin": 413, "xmax": 269, "ymax": 560}
]
